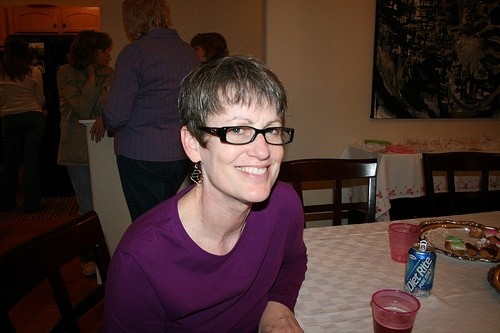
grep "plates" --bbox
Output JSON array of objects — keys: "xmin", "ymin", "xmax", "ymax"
[
  {"xmin": 418, "ymin": 220, "xmax": 500, "ymax": 263},
  {"xmin": 487, "ymin": 264, "xmax": 500, "ymax": 294}
]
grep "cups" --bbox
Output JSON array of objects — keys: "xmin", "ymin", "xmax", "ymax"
[
  {"xmin": 372, "ymin": 289, "xmax": 421, "ymax": 333},
  {"xmin": 389, "ymin": 223, "xmax": 420, "ymax": 263}
]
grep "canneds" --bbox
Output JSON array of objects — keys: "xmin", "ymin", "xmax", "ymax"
[{"xmin": 404, "ymin": 240, "xmax": 436, "ymax": 299}]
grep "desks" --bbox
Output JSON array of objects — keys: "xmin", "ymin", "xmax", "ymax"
[
  {"xmin": 294, "ymin": 212, "xmax": 500, "ymax": 333},
  {"xmin": 340, "ymin": 142, "xmax": 500, "ymax": 224}
]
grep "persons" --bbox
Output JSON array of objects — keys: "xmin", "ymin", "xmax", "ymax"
[
  {"xmin": 190, "ymin": 32, "xmax": 229, "ymax": 62},
  {"xmin": 89, "ymin": 0, "xmax": 201, "ymax": 221},
  {"xmin": 102, "ymin": 55, "xmax": 308, "ymax": 333},
  {"xmin": 57, "ymin": 29, "xmax": 114, "ymax": 216},
  {"xmin": 0, "ymin": 35, "xmax": 46, "ymax": 212}
]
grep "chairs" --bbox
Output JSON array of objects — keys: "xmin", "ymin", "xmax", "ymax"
[
  {"xmin": 276, "ymin": 158, "xmax": 378, "ymax": 228},
  {"xmin": 422, "ymin": 150, "xmax": 500, "ymax": 218},
  {"xmin": 0, "ymin": 211, "xmax": 112, "ymax": 333}
]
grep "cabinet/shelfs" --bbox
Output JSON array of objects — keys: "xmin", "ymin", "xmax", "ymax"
[{"xmin": 11, "ymin": 5, "xmax": 101, "ymax": 35}]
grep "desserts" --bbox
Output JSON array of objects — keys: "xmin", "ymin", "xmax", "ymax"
[{"xmin": 445, "ymin": 226, "xmax": 500, "ymax": 260}]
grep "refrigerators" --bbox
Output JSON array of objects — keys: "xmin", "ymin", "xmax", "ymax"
[{"xmin": 9, "ymin": 34, "xmax": 75, "ymax": 197}]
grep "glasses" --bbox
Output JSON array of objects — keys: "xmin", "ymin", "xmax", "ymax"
[{"xmin": 198, "ymin": 126, "xmax": 294, "ymax": 145}]
